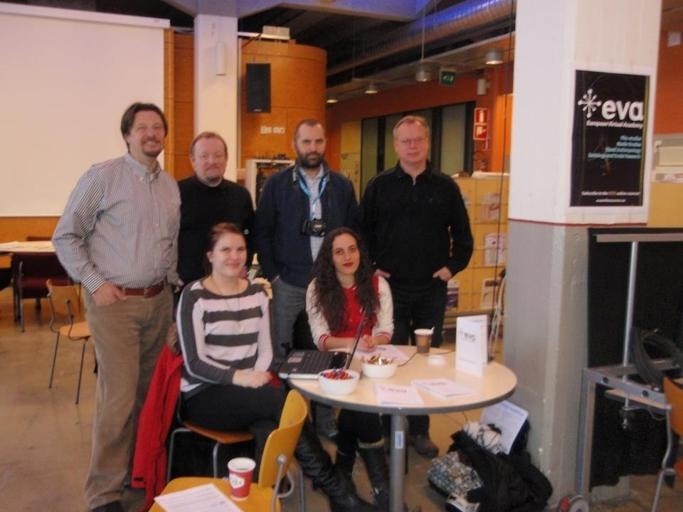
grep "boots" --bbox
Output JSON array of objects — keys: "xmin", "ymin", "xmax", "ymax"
[{"xmin": 294, "ymin": 415, "xmax": 408, "ymax": 512}]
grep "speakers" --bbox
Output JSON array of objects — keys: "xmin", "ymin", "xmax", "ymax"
[{"xmin": 245, "ymin": 63, "xmax": 271, "ymax": 113}]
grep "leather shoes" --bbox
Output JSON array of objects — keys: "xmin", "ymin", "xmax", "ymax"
[{"xmin": 410, "ymin": 434, "xmax": 438, "ymax": 458}]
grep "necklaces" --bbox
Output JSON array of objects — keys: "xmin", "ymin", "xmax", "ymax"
[{"xmin": 212, "ymin": 275, "xmax": 243, "ymax": 331}]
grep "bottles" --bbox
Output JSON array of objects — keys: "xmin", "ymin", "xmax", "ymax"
[{"xmin": 256, "ymin": 168, "xmax": 263, "ymax": 204}]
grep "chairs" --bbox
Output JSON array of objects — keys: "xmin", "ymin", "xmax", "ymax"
[
  {"xmin": 11, "ymin": 253, "xmax": 61, "ymax": 332},
  {"xmin": 25, "ymin": 234, "xmax": 75, "ymax": 287},
  {"xmin": 170, "ymin": 422, "xmax": 306, "ymax": 512},
  {"xmin": 139, "ymin": 387, "xmax": 308, "ymax": 512},
  {"xmin": 651, "ymin": 377, "xmax": 683, "ymax": 511},
  {"xmin": 44, "ymin": 279, "xmax": 93, "ymax": 404}
]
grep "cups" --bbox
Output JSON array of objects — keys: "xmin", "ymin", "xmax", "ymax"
[
  {"xmin": 414, "ymin": 328, "xmax": 433, "ymax": 353},
  {"xmin": 226, "ymin": 457, "xmax": 256, "ymax": 503}
]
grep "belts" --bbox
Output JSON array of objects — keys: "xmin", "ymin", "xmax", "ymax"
[{"xmin": 116, "ymin": 276, "xmax": 167, "ymax": 298}]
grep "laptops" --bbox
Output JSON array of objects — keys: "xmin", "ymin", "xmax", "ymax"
[{"xmin": 278, "ymin": 303, "xmax": 368, "ymax": 379}]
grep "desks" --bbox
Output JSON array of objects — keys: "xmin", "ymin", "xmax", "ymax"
[
  {"xmin": 0, "ymin": 241, "xmax": 57, "ymax": 332},
  {"xmin": 286, "ymin": 343, "xmax": 517, "ymax": 512}
]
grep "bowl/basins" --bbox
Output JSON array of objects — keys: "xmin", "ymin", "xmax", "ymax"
[
  {"xmin": 317, "ymin": 368, "xmax": 361, "ymax": 398},
  {"xmin": 361, "ymin": 355, "xmax": 397, "ymax": 379}
]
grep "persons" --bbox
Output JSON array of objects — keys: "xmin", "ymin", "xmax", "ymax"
[
  {"xmin": 178, "ymin": 221, "xmax": 379, "ymax": 512},
  {"xmin": 256, "ymin": 119, "xmax": 358, "ymax": 442},
  {"xmin": 174, "ymin": 131, "xmax": 256, "ymax": 286},
  {"xmin": 305, "ymin": 227, "xmax": 408, "ymax": 512},
  {"xmin": 358, "ymin": 113, "xmax": 475, "ymax": 459},
  {"xmin": 54, "ymin": 101, "xmax": 182, "ymax": 512}
]
